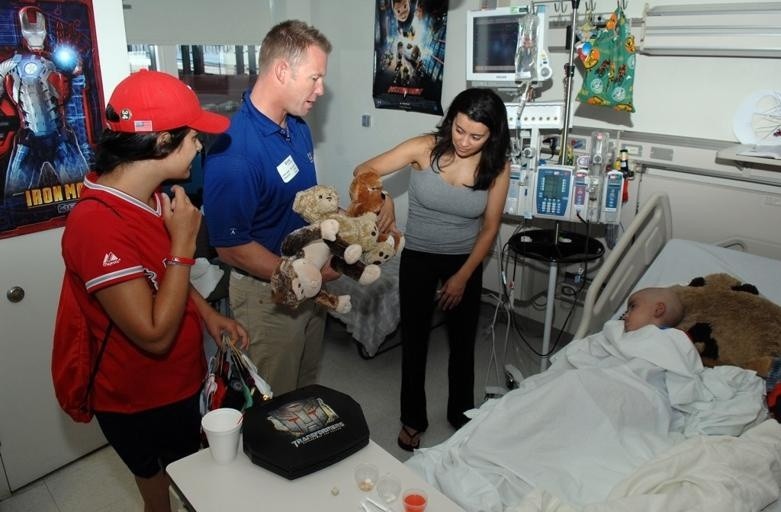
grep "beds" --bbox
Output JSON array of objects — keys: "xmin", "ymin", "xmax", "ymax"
[{"xmin": 404, "ymin": 193, "xmax": 781, "ymax": 512}]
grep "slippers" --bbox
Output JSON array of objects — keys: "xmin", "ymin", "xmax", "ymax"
[{"xmin": 397, "ymin": 424, "xmax": 421, "ymax": 452}]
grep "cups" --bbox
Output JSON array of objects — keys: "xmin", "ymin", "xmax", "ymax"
[
  {"xmin": 403, "ymin": 488, "xmax": 428, "ymax": 512},
  {"xmin": 377, "ymin": 472, "xmax": 401, "ymax": 504},
  {"xmin": 355, "ymin": 463, "xmax": 379, "ymax": 492},
  {"xmin": 201, "ymin": 407, "xmax": 243, "ymax": 463}
]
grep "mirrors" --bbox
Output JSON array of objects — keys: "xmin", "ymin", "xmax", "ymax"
[{"xmin": 127, "ymin": 44, "xmax": 261, "ymax": 104}]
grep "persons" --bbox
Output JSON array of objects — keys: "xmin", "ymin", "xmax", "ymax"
[
  {"xmin": 621, "ymin": 287, "xmax": 687, "ymax": 335},
  {"xmin": 352, "ymin": 87, "xmax": 512, "ymax": 453},
  {"xmin": 202, "ymin": 21, "xmax": 343, "ymax": 405},
  {"xmin": 390, "ymin": 1, "xmax": 420, "ymax": 39},
  {"xmin": 59, "ymin": 67, "xmax": 252, "ymax": 512}
]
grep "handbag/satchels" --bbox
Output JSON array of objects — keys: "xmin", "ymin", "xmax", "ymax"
[{"xmin": 51, "ymin": 197, "xmax": 113, "ymax": 423}]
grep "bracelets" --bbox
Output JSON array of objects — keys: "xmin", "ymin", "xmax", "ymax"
[{"xmin": 165, "ymin": 255, "xmax": 197, "ymax": 265}]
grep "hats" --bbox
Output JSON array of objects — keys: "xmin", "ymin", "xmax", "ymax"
[{"xmin": 105, "ymin": 68, "xmax": 231, "ymax": 135}]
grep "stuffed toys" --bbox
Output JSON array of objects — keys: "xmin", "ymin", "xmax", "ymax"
[
  {"xmin": 347, "ymin": 172, "xmax": 406, "ymax": 257},
  {"xmin": 292, "ymin": 184, "xmax": 396, "ymax": 268},
  {"xmin": 270, "ymin": 219, "xmax": 383, "ymax": 315}
]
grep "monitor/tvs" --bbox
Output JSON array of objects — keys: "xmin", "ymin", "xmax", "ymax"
[{"xmin": 465, "ymin": 5, "xmax": 552, "ymax": 91}]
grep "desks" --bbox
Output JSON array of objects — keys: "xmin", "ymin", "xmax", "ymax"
[{"xmin": 165, "ymin": 438, "xmax": 468, "ymax": 512}]
